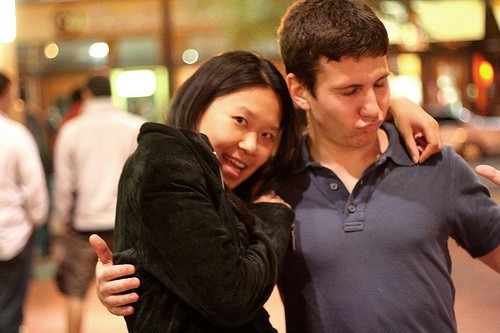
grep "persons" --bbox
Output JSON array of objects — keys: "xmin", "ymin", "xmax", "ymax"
[
  {"xmin": 0, "ymin": 74, "xmax": 153, "ymax": 333},
  {"xmin": 88, "ymin": 0, "xmax": 500, "ymax": 333},
  {"xmin": 111, "ymin": 48, "xmax": 444, "ymax": 332}
]
